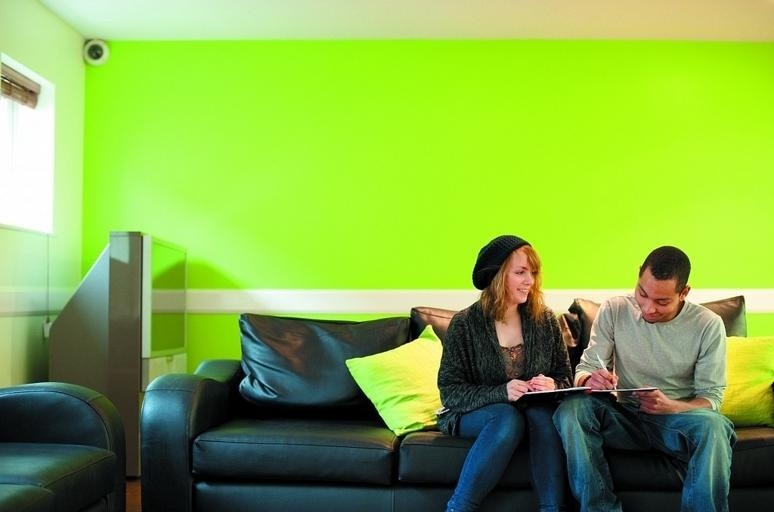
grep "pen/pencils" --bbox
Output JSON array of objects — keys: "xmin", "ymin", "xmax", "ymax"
[{"xmin": 597, "ymin": 354, "xmax": 617, "ymax": 390}]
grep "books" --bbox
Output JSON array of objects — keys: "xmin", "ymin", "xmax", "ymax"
[
  {"xmin": 592, "ymin": 387, "xmax": 658, "ymax": 394},
  {"xmin": 514, "ymin": 386, "xmax": 595, "ymax": 404}
]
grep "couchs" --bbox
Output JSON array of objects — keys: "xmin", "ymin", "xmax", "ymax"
[
  {"xmin": 0, "ymin": 383, "xmax": 126, "ymax": 512},
  {"xmin": 137, "ymin": 311, "xmax": 774, "ymax": 512}
]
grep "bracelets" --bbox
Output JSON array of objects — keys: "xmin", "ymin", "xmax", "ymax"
[{"xmin": 581, "ymin": 375, "xmax": 591, "ymax": 387}]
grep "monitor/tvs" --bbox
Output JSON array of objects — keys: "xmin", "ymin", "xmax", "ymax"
[{"xmin": 141, "ymin": 234, "xmax": 188, "ymax": 358}]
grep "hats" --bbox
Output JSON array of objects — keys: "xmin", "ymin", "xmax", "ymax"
[{"xmin": 472, "ymin": 233, "xmax": 533, "ymax": 291}]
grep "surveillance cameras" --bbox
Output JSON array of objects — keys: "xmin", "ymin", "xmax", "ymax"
[{"xmin": 83, "ymin": 39, "xmax": 109, "ymax": 65}]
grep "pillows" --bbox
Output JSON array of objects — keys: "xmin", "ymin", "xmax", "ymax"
[
  {"xmin": 410, "ymin": 308, "xmax": 583, "ymax": 381},
  {"xmin": 237, "ymin": 313, "xmax": 414, "ymax": 420},
  {"xmin": 568, "ymin": 295, "xmax": 745, "ymax": 405},
  {"xmin": 716, "ymin": 337, "xmax": 774, "ymax": 429},
  {"xmin": 346, "ymin": 326, "xmax": 451, "ymax": 437}
]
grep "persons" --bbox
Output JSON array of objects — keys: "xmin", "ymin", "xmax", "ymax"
[
  {"xmin": 434, "ymin": 234, "xmax": 575, "ymax": 512},
  {"xmin": 552, "ymin": 244, "xmax": 741, "ymax": 512}
]
